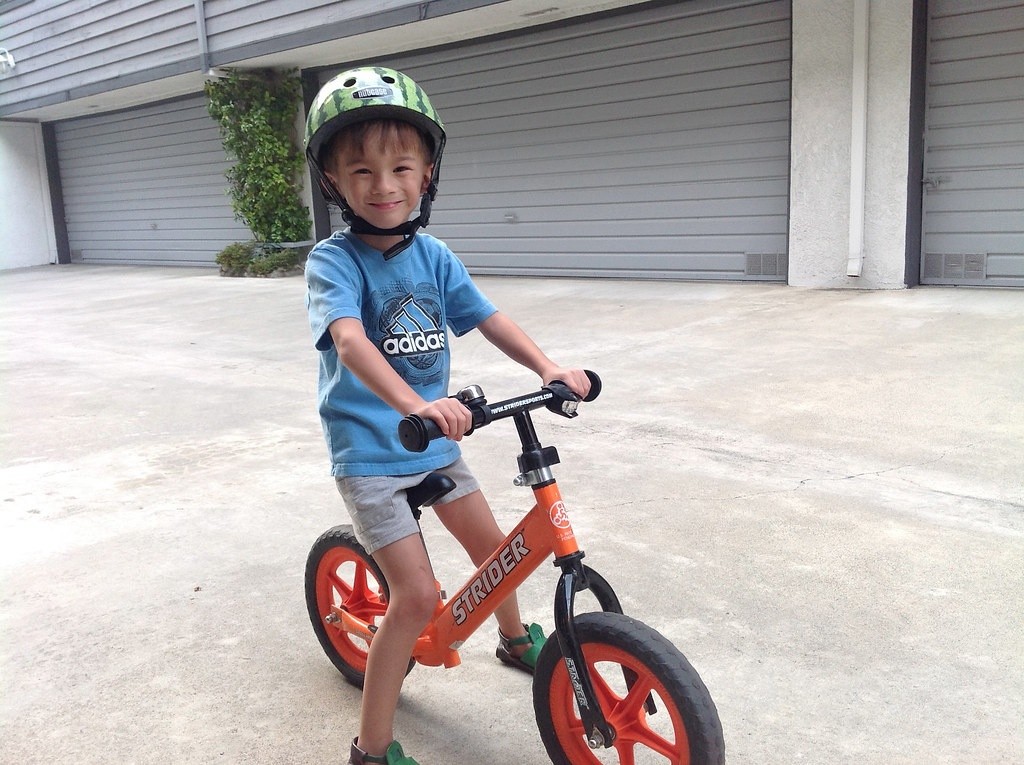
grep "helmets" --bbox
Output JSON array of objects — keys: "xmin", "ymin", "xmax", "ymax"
[{"xmin": 303, "ymin": 66, "xmax": 447, "ymax": 205}]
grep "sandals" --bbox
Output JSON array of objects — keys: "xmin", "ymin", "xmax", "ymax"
[
  {"xmin": 496, "ymin": 622, "xmax": 548, "ymax": 674},
  {"xmin": 347, "ymin": 736, "xmax": 420, "ymax": 764}
]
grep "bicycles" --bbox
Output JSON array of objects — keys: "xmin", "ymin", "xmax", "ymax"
[{"xmin": 303, "ymin": 367, "xmax": 729, "ymax": 765}]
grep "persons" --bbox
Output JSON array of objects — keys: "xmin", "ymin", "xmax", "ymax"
[{"xmin": 300, "ymin": 64, "xmax": 593, "ymax": 764}]
